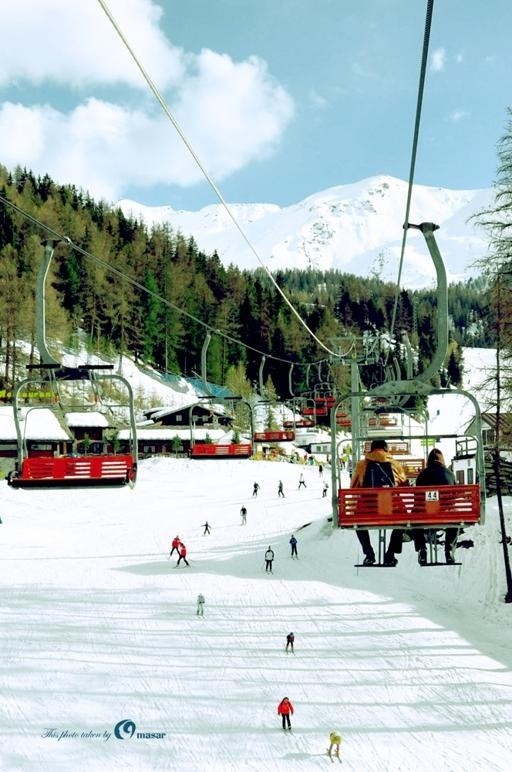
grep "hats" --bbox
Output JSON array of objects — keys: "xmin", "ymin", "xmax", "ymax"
[{"xmin": 427, "ymin": 449, "xmax": 445, "ymax": 464}]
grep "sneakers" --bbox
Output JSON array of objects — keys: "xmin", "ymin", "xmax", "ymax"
[
  {"xmin": 446, "ymin": 550, "xmax": 456, "ymax": 563},
  {"xmin": 417, "ymin": 550, "xmax": 427, "ymax": 564},
  {"xmin": 363, "ymin": 555, "xmax": 376, "ymax": 565},
  {"xmin": 384, "ymin": 553, "xmax": 398, "ymax": 564}
]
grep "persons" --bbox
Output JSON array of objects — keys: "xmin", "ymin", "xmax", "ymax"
[
  {"xmin": 394, "ymin": 445, "xmax": 421, "ymax": 474},
  {"xmin": 265, "ymin": 545, "xmax": 275, "ymax": 575},
  {"xmin": 170, "ymin": 536, "xmax": 180, "ymax": 556},
  {"xmin": 322, "ymin": 480, "xmax": 328, "ymax": 497},
  {"xmin": 270, "ymin": 444, "xmax": 352, "ymax": 480},
  {"xmin": 290, "ymin": 534, "xmax": 299, "ymax": 560},
  {"xmin": 277, "ymin": 480, "xmax": 285, "ymax": 497},
  {"xmin": 328, "ymin": 728, "xmax": 341, "ymax": 759},
  {"xmin": 194, "ymin": 593, "xmax": 204, "ymax": 616},
  {"xmin": 240, "ymin": 505, "xmax": 247, "ymax": 525},
  {"xmin": 252, "ymin": 482, "xmax": 260, "ymax": 497},
  {"xmin": 277, "ymin": 696, "xmax": 294, "ymax": 733},
  {"xmin": 285, "ymin": 631, "xmax": 296, "ymax": 654},
  {"xmin": 175, "ymin": 542, "xmax": 189, "ymax": 566},
  {"xmin": 411, "ymin": 449, "xmax": 458, "ymax": 563},
  {"xmin": 202, "ymin": 521, "xmax": 212, "ymax": 536},
  {"xmin": 297, "ymin": 473, "xmax": 307, "ymax": 488},
  {"xmin": 350, "ymin": 438, "xmax": 410, "ymax": 565}
]
